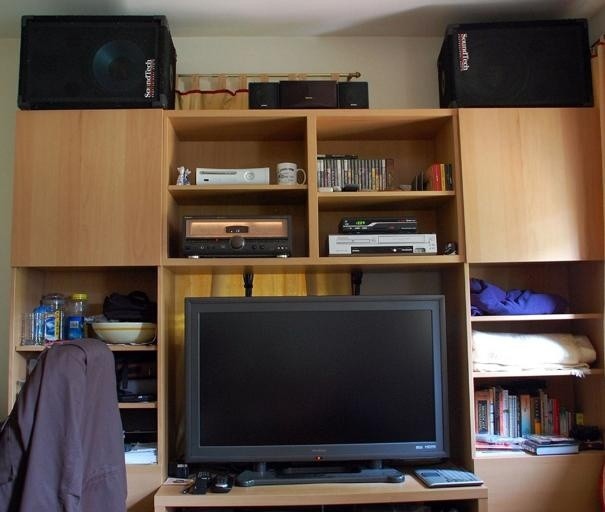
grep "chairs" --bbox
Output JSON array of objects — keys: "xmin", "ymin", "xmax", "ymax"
[{"xmin": 0, "ymin": 337, "xmax": 129, "ymax": 512}]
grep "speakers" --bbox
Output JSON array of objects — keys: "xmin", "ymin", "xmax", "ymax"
[
  {"xmin": 280, "ymin": 79, "xmax": 337, "ymax": 110},
  {"xmin": 338, "ymin": 82, "xmax": 369, "ymax": 109},
  {"xmin": 248, "ymin": 81, "xmax": 278, "ymax": 110}
]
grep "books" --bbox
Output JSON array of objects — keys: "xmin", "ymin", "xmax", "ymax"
[
  {"xmin": 316, "ymin": 154, "xmax": 454, "ymax": 193},
  {"xmin": 125, "ymin": 440, "xmax": 159, "ymax": 466},
  {"xmin": 472, "ymin": 383, "xmax": 604, "ymax": 458}
]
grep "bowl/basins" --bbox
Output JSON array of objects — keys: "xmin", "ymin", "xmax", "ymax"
[{"xmin": 88, "ymin": 321, "xmax": 157, "ymax": 344}]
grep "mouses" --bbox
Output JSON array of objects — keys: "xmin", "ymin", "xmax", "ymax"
[{"xmin": 213, "ymin": 473, "xmax": 233, "ymax": 494}]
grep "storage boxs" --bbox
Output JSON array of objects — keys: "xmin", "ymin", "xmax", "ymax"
[
  {"xmin": 245, "ymin": 80, "xmax": 280, "ymax": 109},
  {"xmin": 274, "ymin": 77, "xmax": 339, "ymax": 108},
  {"xmin": 337, "ymin": 80, "xmax": 372, "ymax": 112}
]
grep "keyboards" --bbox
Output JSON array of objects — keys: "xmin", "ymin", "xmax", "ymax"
[{"xmin": 412, "ymin": 464, "xmax": 483, "ymax": 488}]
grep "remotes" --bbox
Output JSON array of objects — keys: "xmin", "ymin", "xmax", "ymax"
[{"xmin": 196, "ymin": 471, "xmax": 211, "ymax": 487}]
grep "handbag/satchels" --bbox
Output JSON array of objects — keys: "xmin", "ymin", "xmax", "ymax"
[{"xmin": 103, "ymin": 290, "xmax": 156, "ymax": 322}]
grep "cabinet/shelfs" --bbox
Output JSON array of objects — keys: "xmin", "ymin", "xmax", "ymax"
[
  {"xmin": 155, "ymin": 103, "xmax": 491, "ymax": 512},
  {"xmin": 6, "ymin": 105, "xmax": 166, "ymax": 512},
  {"xmin": 444, "ymin": 103, "xmax": 605, "ymax": 512}
]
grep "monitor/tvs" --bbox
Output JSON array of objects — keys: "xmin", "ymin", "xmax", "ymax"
[{"xmin": 185, "ymin": 295, "xmax": 450, "ymax": 486}]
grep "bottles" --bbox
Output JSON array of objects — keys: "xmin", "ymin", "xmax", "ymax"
[{"xmin": 20, "ymin": 287, "xmax": 90, "ymax": 345}]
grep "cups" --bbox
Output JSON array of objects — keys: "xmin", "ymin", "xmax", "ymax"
[{"xmin": 274, "ymin": 162, "xmax": 307, "ymax": 186}]
